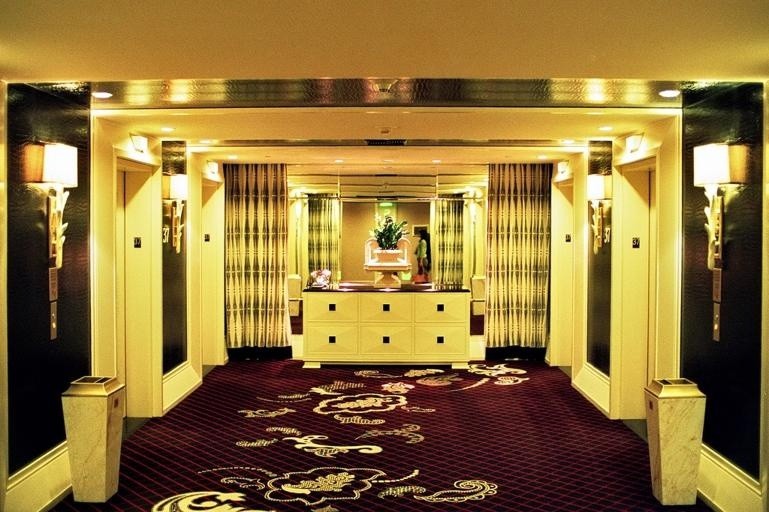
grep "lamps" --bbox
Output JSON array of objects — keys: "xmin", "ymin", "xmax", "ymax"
[
  {"xmin": 42, "ymin": 141, "xmax": 81, "ymax": 269},
  {"xmin": 169, "ymin": 172, "xmax": 190, "ymax": 256},
  {"xmin": 693, "ymin": 144, "xmax": 731, "ymax": 271},
  {"xmin": 586, "ymin": 174, "xmax": 606, "ymax": 256}
]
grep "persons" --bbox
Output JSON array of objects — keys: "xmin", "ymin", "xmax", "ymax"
[{"xmin": 414, "ymin": 230, "xmax": 427, "ymax": 276}]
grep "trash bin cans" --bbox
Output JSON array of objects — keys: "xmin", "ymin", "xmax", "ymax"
[
  {"xmin": 61, "ymin": 376, "xmax": 127, "ymax": 503},
  {"xmin": 643, "ymin": 378, "xmax": 707, "ymax": 505}
]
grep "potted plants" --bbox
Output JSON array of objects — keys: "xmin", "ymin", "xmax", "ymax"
[{"xmin": 364, "ymin": 213, "xmax": 415, "ymax": 289}]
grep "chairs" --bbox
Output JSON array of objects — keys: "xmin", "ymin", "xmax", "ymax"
[
  {"xmin": 288, "ymin": 276, "xmax": 304, "ymax": 324},
  {"xmin": 470, "ymin": 278, "xmax": 485, "ymax": 320}
]
